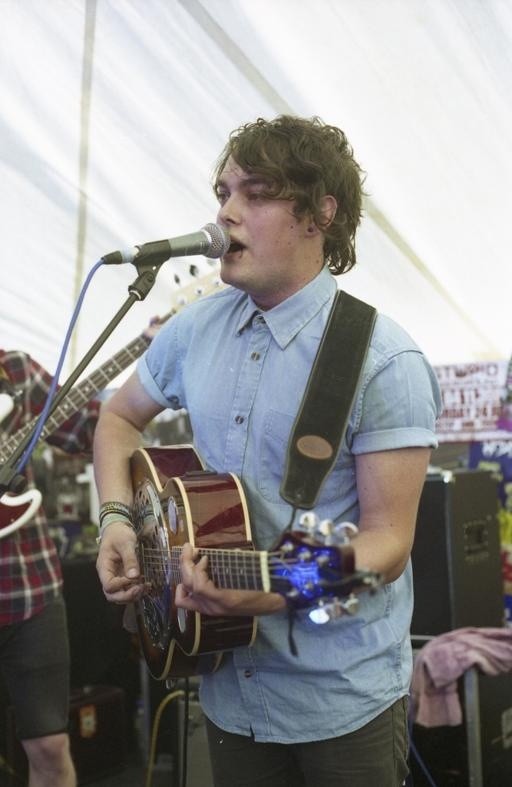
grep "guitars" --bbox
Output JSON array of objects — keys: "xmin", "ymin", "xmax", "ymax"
[
  {"xmin": 1, "ymin": 258, "xmax": 230, "ymax": 468},
  {"xmin": 131, "ymin": 443, "xmax": 384, "ymax": 681}
]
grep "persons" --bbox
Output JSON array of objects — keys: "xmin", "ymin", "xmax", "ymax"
[
  {"xmin": 0, "ymin": 305, "xmax": 165, "ymax": 785},
  {"xmin": 92, "ymin": 118, "xmax": 435, "ymax": 785}
]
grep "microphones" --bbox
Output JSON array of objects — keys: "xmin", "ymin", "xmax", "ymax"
[{"xmin": 102, "ymin": 222, "xmax": 232, "ymax": 264}]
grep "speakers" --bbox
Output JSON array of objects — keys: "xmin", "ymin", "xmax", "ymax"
[
  {"xmin": 8, "ymin": 681, "xmax": 129, "ymax": 787},
  {"xmin": 411, "ymin": 468, "xmax": 506, "ymax": 648}
]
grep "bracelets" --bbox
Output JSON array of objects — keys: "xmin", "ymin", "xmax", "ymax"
[{"xmin": 95, "ymin": 500, "xmax": 137, "ymax": 545}]
